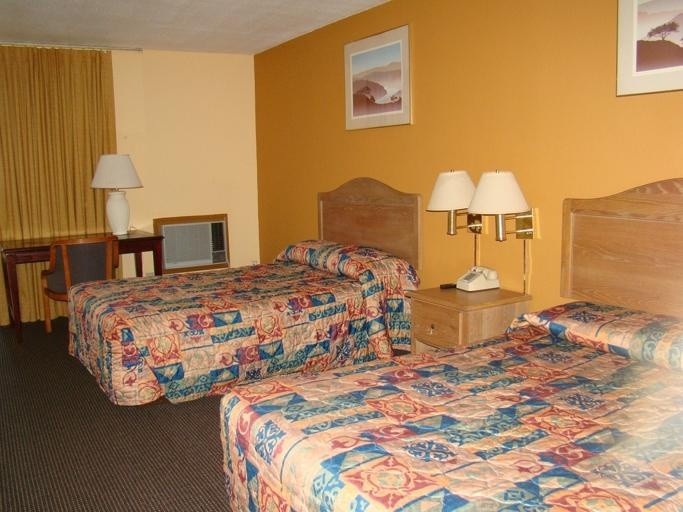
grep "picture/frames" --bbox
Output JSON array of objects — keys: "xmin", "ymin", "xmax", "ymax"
[
  {"xmin": 341, "ymin": 23, "xmax": 413, "ymax": 133},
  {"xmin": 612, "ymin": 0, "xmax": 682, "ymax": 100}
]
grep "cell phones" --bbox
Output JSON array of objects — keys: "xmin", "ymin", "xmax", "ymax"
[{"xmin": 440, "ymin": 283, "xmax": 456, "ymax": 289}]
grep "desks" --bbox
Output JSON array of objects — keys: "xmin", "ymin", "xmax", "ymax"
[{"xmin": 2, "ymin": 232, "xmax": 170, "ymax": 343}]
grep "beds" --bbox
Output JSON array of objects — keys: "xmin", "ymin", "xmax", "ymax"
[
  {"xmin": 212, "ymin": 175, "xmax": 682, "ymax": 511},
  {"xmin": 66, "ymin": 177, "xmax": 429, "ymax": 410}
]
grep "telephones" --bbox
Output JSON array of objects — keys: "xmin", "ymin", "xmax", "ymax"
[{"xmin": 456, "ymin": 265, "xmax": 500, "ymax": 292}]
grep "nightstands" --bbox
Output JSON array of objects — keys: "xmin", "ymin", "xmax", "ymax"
[{"xmin": 410, "ymin": 286, "xmax": 536, "ymax": 359}]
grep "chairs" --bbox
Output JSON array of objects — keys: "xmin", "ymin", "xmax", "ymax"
[{"xmin": 39, "ymin": 236, "xmax": 120, "ymax": 332}]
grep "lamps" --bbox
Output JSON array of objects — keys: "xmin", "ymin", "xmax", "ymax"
[
  {"xmin": 89, "ymin": 154, "xmax": 144, "ymax": 236},
  {"xmin": 422, "ymin": 166, "xmax": 527, "ymax": 296}
]
